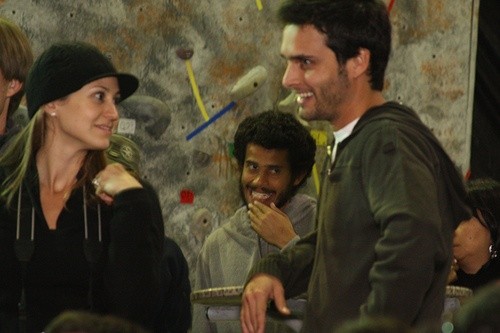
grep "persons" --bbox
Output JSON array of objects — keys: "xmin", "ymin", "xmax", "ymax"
[
  {"xmin": 446, "ymin": 177, "xmax": 500, "ymax": 293},
  {"xmin": 0, "ymin": 18, "xmax": 192, "ymax": 333},
  {"xmin": 191, "ymin": 108, "xmax": 318, "ymax": 333},
  {"xmin": 239, "ymin": 0, "xmax": 474, "ymax": 333}
]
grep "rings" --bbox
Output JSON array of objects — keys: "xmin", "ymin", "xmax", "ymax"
[{"xmin": 91, "ymin": 177, "xmax": 100, "ymax": 186}]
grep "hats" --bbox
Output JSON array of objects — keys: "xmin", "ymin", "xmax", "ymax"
[{"xmin": 25, "ymin": 40, "xmax": 140, "ymax": 120}]
